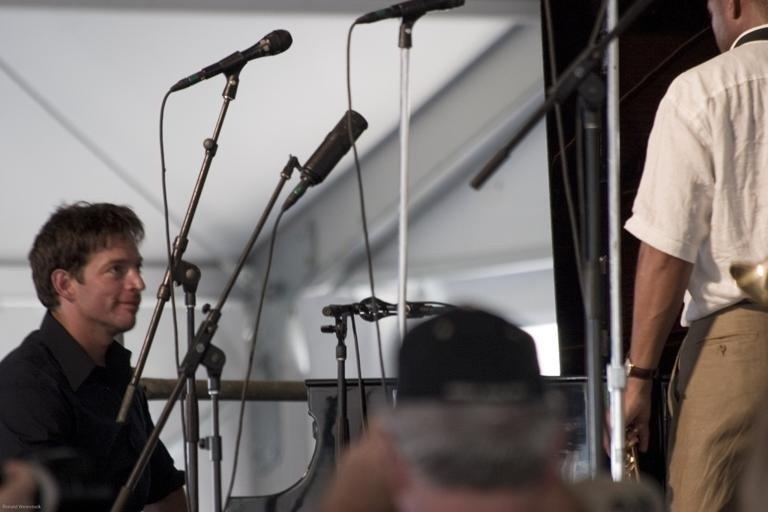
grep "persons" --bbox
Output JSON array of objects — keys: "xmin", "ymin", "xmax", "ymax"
[
  {"xmin": 0, "ymin": 196, "xmax": 189, "ymax": 511},
  {"xmin": 318, "ymin": 307, "xmax": 665, "ymax": 512},
  {"xmin": 599, "ymin": 0, "xmax": 768, "ymax": 512}
]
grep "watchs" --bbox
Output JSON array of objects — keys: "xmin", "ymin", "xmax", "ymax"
[{"xmin": 624, "ymin": 357, "xmax": 657, "ymax": 381}]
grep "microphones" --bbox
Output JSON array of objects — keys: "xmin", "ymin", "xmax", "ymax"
[
  {"xmin": 357, "ymin": 0, "xmax": 464, "ymax": 24},
  {"xmin": 170, "ymin": 30, "xmax": 292, "ymax": 91},
  {"xmin": 284, "ymin": 110, "xmax": 367, "ymax": 210}
]
